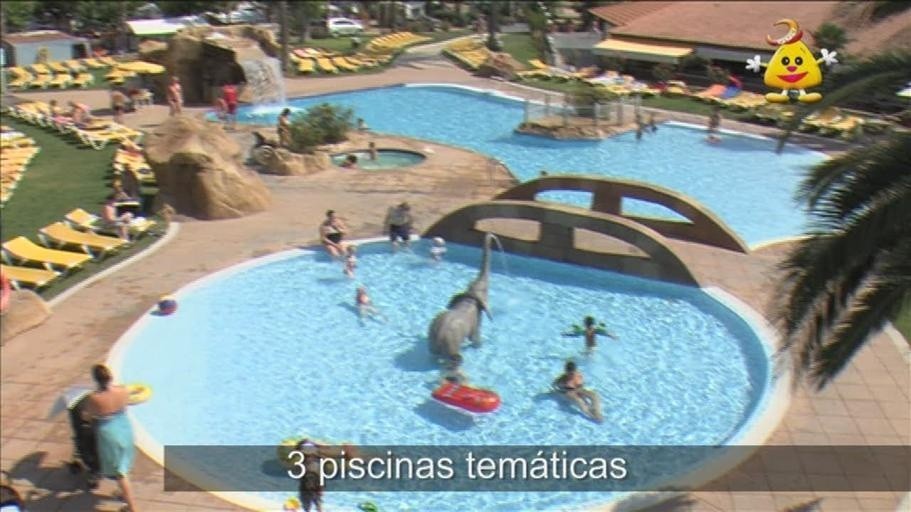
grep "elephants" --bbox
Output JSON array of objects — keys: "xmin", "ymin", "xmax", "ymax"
[{"xmin": 428, "ymin": 231, "xmax": 494, "ymax": 360}]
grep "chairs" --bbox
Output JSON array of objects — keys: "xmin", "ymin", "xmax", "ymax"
[
  {"xmin": 286, "ymin": 30, "xmax": 435, "ymax": 75},
  {"xmin": 1, "ymin": 57, "xmax": 168, "ymax": 292}
]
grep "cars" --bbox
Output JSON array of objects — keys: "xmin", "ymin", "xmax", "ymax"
[{"xmin": 309, "ymin": 16, "xmax": 361, "ymax": 38}]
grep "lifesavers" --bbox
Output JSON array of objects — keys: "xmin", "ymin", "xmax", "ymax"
[
  {"xmin": 432, "ymin": 380, "xmax": 500, "ymax": 412},
  {"xmin": 123, "ymin": 383, "xmax": 152, "ymax": 404}
]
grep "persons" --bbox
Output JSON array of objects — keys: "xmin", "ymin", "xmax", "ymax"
[
  {"xmin": 224, "ymin": 82, "xmax": 237, "ymax": 127},
  {"xmin": 111, "ymin": 89, "xmax": 126, "ymax": 121},
  {"xmin": 102, "ymin": 200, "xmax": 135, "ymax": 243},
  {"xmin": 381, "ymin": 200, "xmax": 414, "ymax": 251},
  {"xmin": 358, "ymin": 118, "xmax": 369, "ymax": 132},
  {"xmin": 563, "ymin": 316, "xmax": 618, "ymax": 349},
  {"xmin": 552, "ymin": 362, "xmax": 603, "ymax": 424},
  {"xmin": 320, "ymin": 210, "xmax": 350, "ymax": 257},
  {"xmin": 367, "ymin": 144, "xmax": 378, "ymax": 164},
  {"xmin": 78, "ymin": 367, "xmax": 140, "ymax": 512},
  {"xmin": 296, "ymin": 442, "xmax": 325, "ymax": 512},
  {"xmin": 356, "ymin": 286, "xmax": 384, "ymax": 325},
  {"xmin": 42, "ymin": 95, "xmax": 93, "ymax": 131},
  {"xmin": 342, "ymin": 155, "xmax": 360, "ymax": 169},
  {"xmin": 342, "ymin": 246, "xmax": 358, "ymax": 277},
  {"xmin": 211, "ymin": 95, "xmax": 227, "ymax": 121},
  {"xmin": 165, "ymin": 76, "xmax": 186, "ymax": 114},
  {"xmin": 276, "ymin": 108, "xmax": 296, "ymax": 145}
]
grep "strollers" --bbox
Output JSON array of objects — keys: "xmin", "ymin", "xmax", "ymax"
[{"xmin": 62, "ymin": 388, "xmax": 111, "ymax": 490}]
